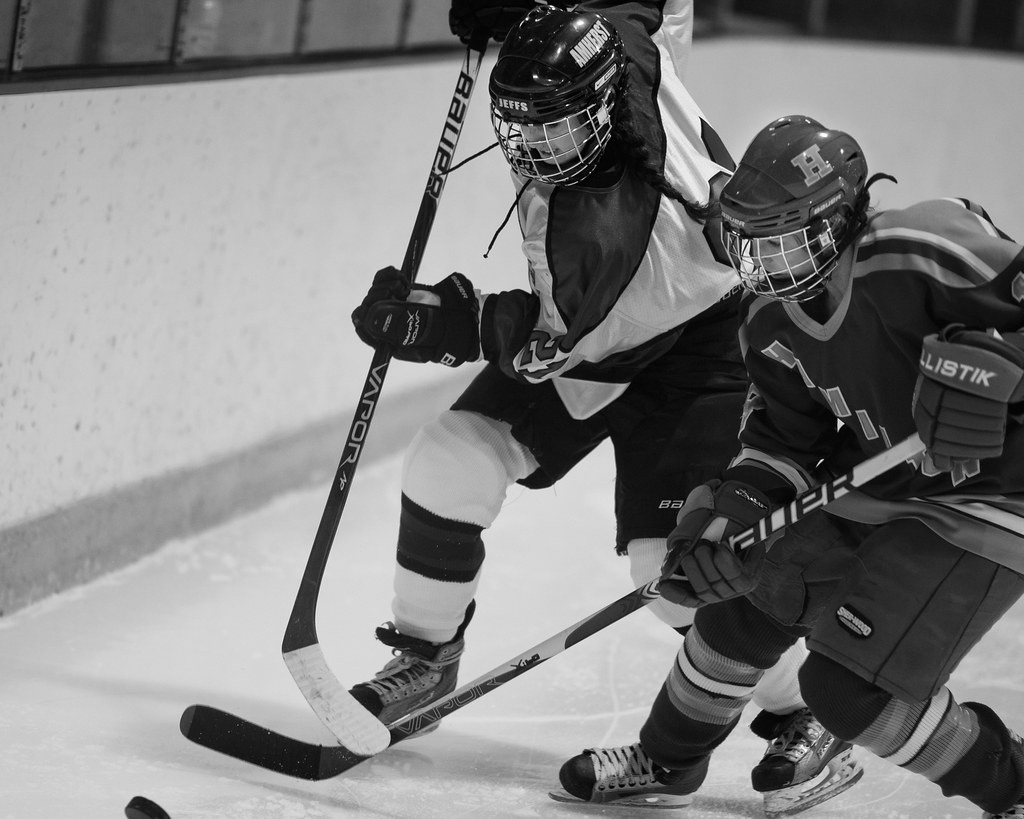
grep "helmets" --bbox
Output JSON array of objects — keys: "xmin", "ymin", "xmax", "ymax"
[
  {"xmin": 722, "ymin": 114, "xmax": 870, "ymax": 300},
  {"xmin": 489, "ymin": 5, "xmax": 624, "ymax": 184}
]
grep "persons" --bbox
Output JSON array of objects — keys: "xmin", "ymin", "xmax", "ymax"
[
  {"xmin": 548, "ymin": 111, "xmax": 1024, "ymax": 819},
  {"xmin": 339, "ymin": 1, "xmax": 869, "ymax": 819}
]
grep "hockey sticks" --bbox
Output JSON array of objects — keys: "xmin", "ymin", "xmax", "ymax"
[
  {"xmin": 179, "ymin": 431, "xmax": 927, "ymax": 781},
  {"xmin": 282, "ymin": 36, "xmax": 489, "ymax": 756}
]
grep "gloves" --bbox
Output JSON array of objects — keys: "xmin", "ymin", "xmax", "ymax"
[
  {"xmin": 350, "ymin": 265, "xmax": 480, "ymax": 368},
  {"xmin": 449, "ymin": 0, "xmax": 548, "ymax": 51},
  {"xmin": 656, "ymin": 477, "xmax": 786, "ymax": 607},
  {"xmin": 911, "ymin": 330, "xmax": 1021, "ymax": 467}
]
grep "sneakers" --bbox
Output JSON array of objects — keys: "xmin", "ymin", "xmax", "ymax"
[
  {"xmin": 752, "ymin": 707, "xmax": 864, "ymax": 815},
  {"xmin": 550, "ymin": 741, "xmax": 710, "ymax": 810},
  {"xmin": 347, "ymin": 640, "xmax": 464, "ymax": 734}
]
